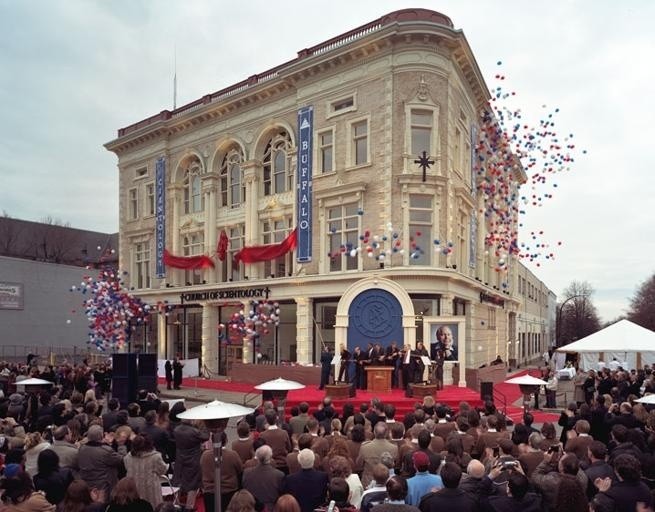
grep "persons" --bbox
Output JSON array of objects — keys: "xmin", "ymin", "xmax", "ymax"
[
  {"xmin": 532, "ymin": 358, "xmax": 655, "ymax": 511},
  {"xmin": 430, "ymin": 323, "xmax": 458, "ymax": 358},
  {"xmin": 543, "ymin": 350, "xmax": 553, "ymax": 366},
  {"xmin": 101, "ymin": 390, "xmax": 558, "ymax": 511},
  {"xmin": 319, "ymin": 342, "xmax": 445, "ymax": 392},
  {"xmin": 165, "ymin": 356, "xmax": 184, "ymax": 390},
  {"xmin": 493, "ymin": 355, "xmax": 503, "ymax": 365},
  {"xmin": 1, "ymin": 351, "xmax": 118, "ymax": 511}
]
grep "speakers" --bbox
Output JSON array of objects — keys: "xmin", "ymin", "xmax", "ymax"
[
  {"xmin": 137, "ymin": 378, "xmax": 157, "ymax": 400},
  {"xmin": 112, "ymin": 353, "xmax": 136, "ymax": 379},
  {"xmin": 481, "ymin": 382, "xmax": 492, "ymax": 399},
  {"xmin": 138, "ymin": 354, "xmax": 158, "ymax": 378},
  {"xmin": 112, "ymin": 379, "xmax": 136, "ymax": 409}
]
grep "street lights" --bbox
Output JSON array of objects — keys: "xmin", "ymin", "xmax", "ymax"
[{"xmin": 557, "ymin": 292, "xmax": 591, "ymax": 350}]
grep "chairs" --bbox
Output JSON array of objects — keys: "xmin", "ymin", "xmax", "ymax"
[{"xmin": 160, "ymin": 475, "xmax": 181, "ymax": 505}]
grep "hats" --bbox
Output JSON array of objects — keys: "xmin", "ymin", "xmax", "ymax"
[{"xmin": 412, "ymin": 451, "xmax": 430, "ymax": 468}]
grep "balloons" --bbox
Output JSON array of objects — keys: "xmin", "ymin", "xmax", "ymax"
[
  {"xmin": 217, "ymin": 299, "xmax": 282, "ymax": 344},
  {"xmin": 66, "ymin": 245, "xmax": 152, "ymax": 350},
  {"xmin": 328, "ymin": 207, "xmax": 453, "ymax": 265},
  {"xmin": 473, "ymin": 60, "xmax": 587, "ymax": 291}
]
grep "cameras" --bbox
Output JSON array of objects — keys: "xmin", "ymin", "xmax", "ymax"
[
  {"xmin": 503, "ymin": 461, "xmax": 517, "ymax": 469},
  {"xmin": 549, "ymin": 445, "xmax": 559, "ymax": 453}
]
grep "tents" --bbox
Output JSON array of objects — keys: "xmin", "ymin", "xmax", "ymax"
[{"xmin": 552, "ymin": 319, "xmax": 655, "ymax": 371}]
